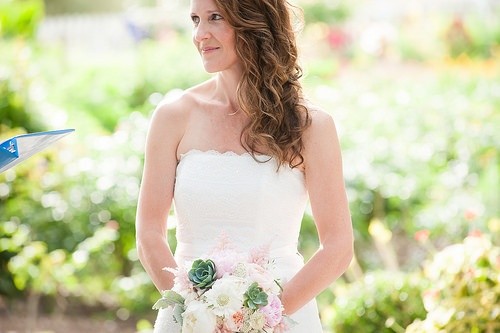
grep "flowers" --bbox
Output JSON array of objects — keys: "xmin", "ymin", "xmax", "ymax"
[{"xmin": 152, "ymin": 232, "xmax": 298, "ymax": 333}]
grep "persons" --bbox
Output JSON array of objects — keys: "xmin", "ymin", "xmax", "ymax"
[{"xmin": 134, "ymin": 1, "xmax": 354, "ymax": 333}]
